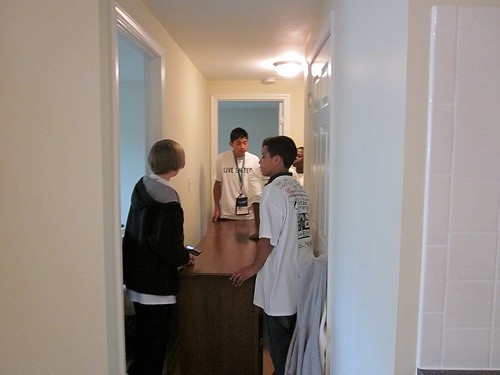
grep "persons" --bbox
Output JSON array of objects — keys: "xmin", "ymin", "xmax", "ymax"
[
  {"xmin": 294, "ymin": 147, "xmax": 305, "ymax": 186},
  {"xmin": 230, "ymin": 136, "xmax": 314, "ymax": 375},
  {"xmin": 212, "ymin": 127, "xmax": 260, "ymax": 221},
  {"xmin": 247, "ymin": 137, "xmax": 300, "ymax": 240},
  {"xmin": 122, "ymin": 140, "xmax": 195, "ymax": 375}
]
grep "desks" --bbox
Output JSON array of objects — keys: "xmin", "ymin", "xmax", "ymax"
[{"xmin": 176, "ymin": 220, "xmax": 265, "ymax": 375}]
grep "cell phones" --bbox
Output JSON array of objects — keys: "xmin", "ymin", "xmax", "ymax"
[{"xmin": 185, "ymin": 244, "xmax": 202, "ymax": 256}]
